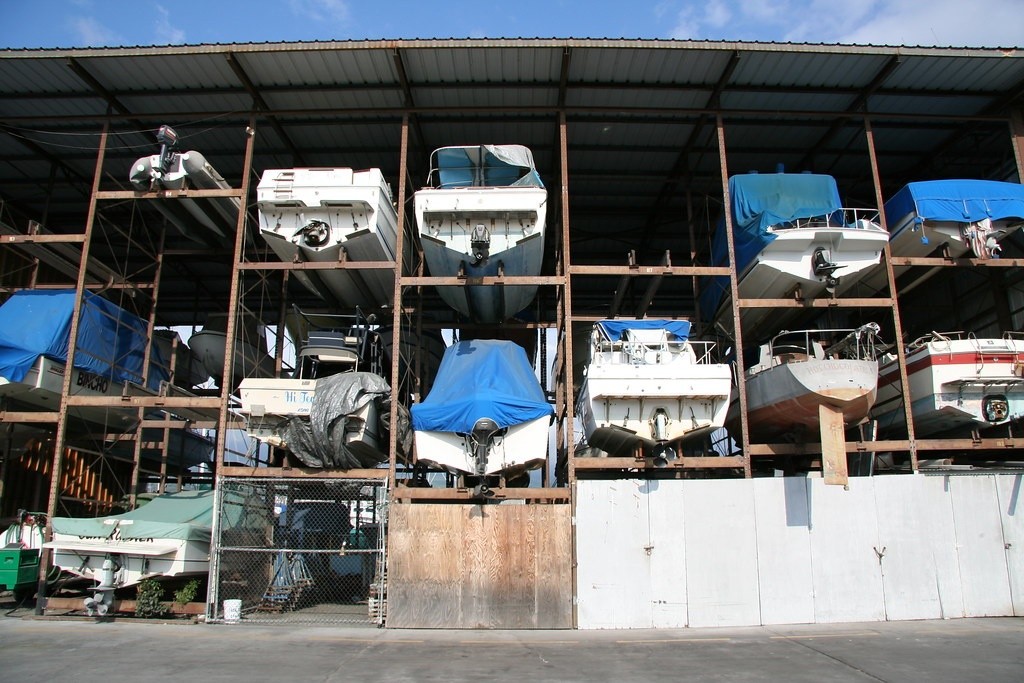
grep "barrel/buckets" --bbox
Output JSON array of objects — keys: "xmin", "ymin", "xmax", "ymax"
[{"xmin": 223, "ymin": 599, "xmax": 242, "ymax": 625}]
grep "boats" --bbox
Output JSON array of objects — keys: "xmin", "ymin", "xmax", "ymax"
[
  {"xmin": 413, "ymin": 145, "xmax": 547, "ymax": 324},
  {"xmin": 704, "ymin": 170, "xmax": 891, "ymax": 342},
  {"xmin": 576, "ymin": 319, "xmax": 732, "ymax": 455},
  {"xmin": 130, "ymin": 123, "xmax": 260, "ymax": 256},
  {"xmin": 408, "ymin": 339, "xmax": 555, "ymax": 476},
  {"xmin": 0, "ymin": 290, "xmax": 209, "ymax": 432},
  {"xmin": 0, "ymin": 491, "xmax": 279, "ymax": 617},
  {"xmin": 813, "ymin": 179, "xmax": 1024, "ymax": 329},
  {"xmin": 724, "ymin": 344, "xmax": 879, "ymax": 451},
  {"xmin": 844, "ymin": 326, "xmax": 1024, "ymax": 441},
  {"xmin": 186, "ymin": 318, "xmax": 291, "ymax": 397},
  {"xmin": 238, "ymin": 303, "xmax": 388, "ymax": 467},
  {"xmin": 257, "ymin": 167, "xmax": 411, "ymax": 311}
]
